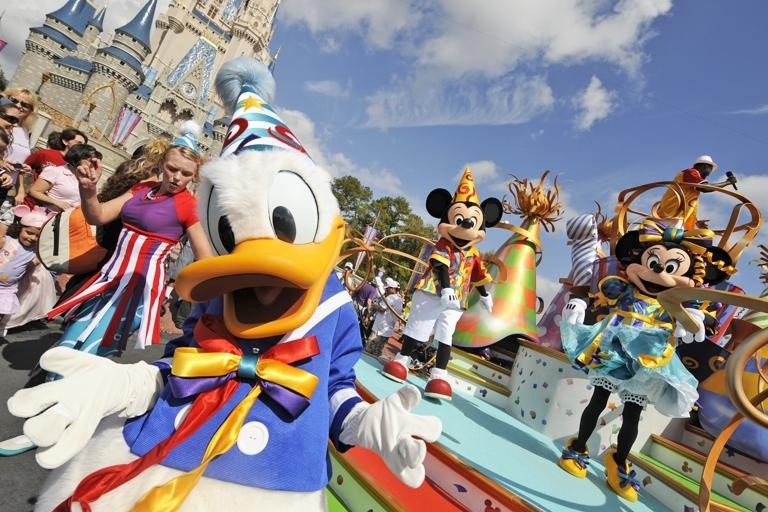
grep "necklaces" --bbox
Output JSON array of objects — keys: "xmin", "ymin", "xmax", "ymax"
[{"xmin": 145, "ymin": 187, "xmax": 164, "ymax": 201}]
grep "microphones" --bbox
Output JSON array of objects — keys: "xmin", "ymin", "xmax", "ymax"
[{"xmin": 725, "ymin": 170, "xmax": 739, "ymax": 192}]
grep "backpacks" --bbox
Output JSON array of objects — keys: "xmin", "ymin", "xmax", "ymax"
[{"xmin": 37, "ymin": 206, "xmax": 106, "ymax": 275}]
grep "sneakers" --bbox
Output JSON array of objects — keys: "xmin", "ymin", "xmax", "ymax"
[{"xmin": 0, "ymin": 435, "xmax": 38, "ymax": 457}]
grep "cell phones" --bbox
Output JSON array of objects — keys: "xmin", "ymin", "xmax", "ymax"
[{"xmin": 12, "ymin": 164, "xmax": 22, "ymax": 169}]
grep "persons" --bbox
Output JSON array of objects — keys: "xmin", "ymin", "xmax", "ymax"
[
  {"xmin": 0, "ymin": 100, "xmax": 20, "ymax": 191},
  {"xmin": 353, "ymin": 277, "xmax": 382, "ymax": 325},
  {"xmin": 45, "ymin": 131, "xmax": 66, "ymax": 153},
  {"xmin": 336, "ymin": 261, "xmax": 354, "ymax": 294},
  {"xmin": 51, "ymin": 137, "xmax": 168, "ymax": 320},
  {"xmin": 0, "ymin": 143, "xmax": 214, "ymax": 458},
  {"xmin": 0, "ymin": 118, "xmax": 15, "ymax": 208},
  {"xmin": 14, "ymin": 127, "xmax": 90, "ymax": 221},
  {"xmin": 0, "ymin": 83, "xmax": 39, "ymax": 190},
  {"xmin": 0, "ymin": 204, "xmax": 59, "ymax": 347},
  {"xmin": 365, "ymin": 279, "xmax": 404, "ymax": 357},
  {"xmin": 364, "ymin": 276, "xmax": 394, "ymax": 343},
  {"xmin": 658, "ymin": 155, "xmax": 738, "ymax": 235},
  {"xmin": 0, "ymin": 143, "xmax": 104, "ymax": 338}
]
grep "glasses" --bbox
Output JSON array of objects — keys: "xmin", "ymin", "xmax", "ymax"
[
  {"xmin": 1, "ymin": 115, "xmax": 19, "ymax": 125},
  {"xmin": 10, "ymin": 97, "xmax": 33, "ymax": 109}
]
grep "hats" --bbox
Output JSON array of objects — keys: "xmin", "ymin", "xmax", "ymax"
[
  {"xmin": 13, "ymin": 204, "xmax": 58, "ymax": 229},
  {"xmin": 171, "ymin": 119, "xmax": 200, "ymax": 152},
  {"xmin": 371, "ymin": 278, "xmax": 380, "ymax": 286},
  {"xmin": 344, "ymin": 262, "xmax": 353, "ymax": 269},
  {"xmin": 384, "ymin": 281, "xmax": 400, "ymax": 289}
]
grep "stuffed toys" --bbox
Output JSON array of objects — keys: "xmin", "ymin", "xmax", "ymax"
[
  {"xmin": 7, "ymin": 54, "xmax": 446, "ymax": 512},
  {"xmin": 381, "ymin": 164, "xmax": 504, "ymax": 401},
  {"xmin": 556, "ymin": 216, "xmax": 739, "ymax": 503}
]
